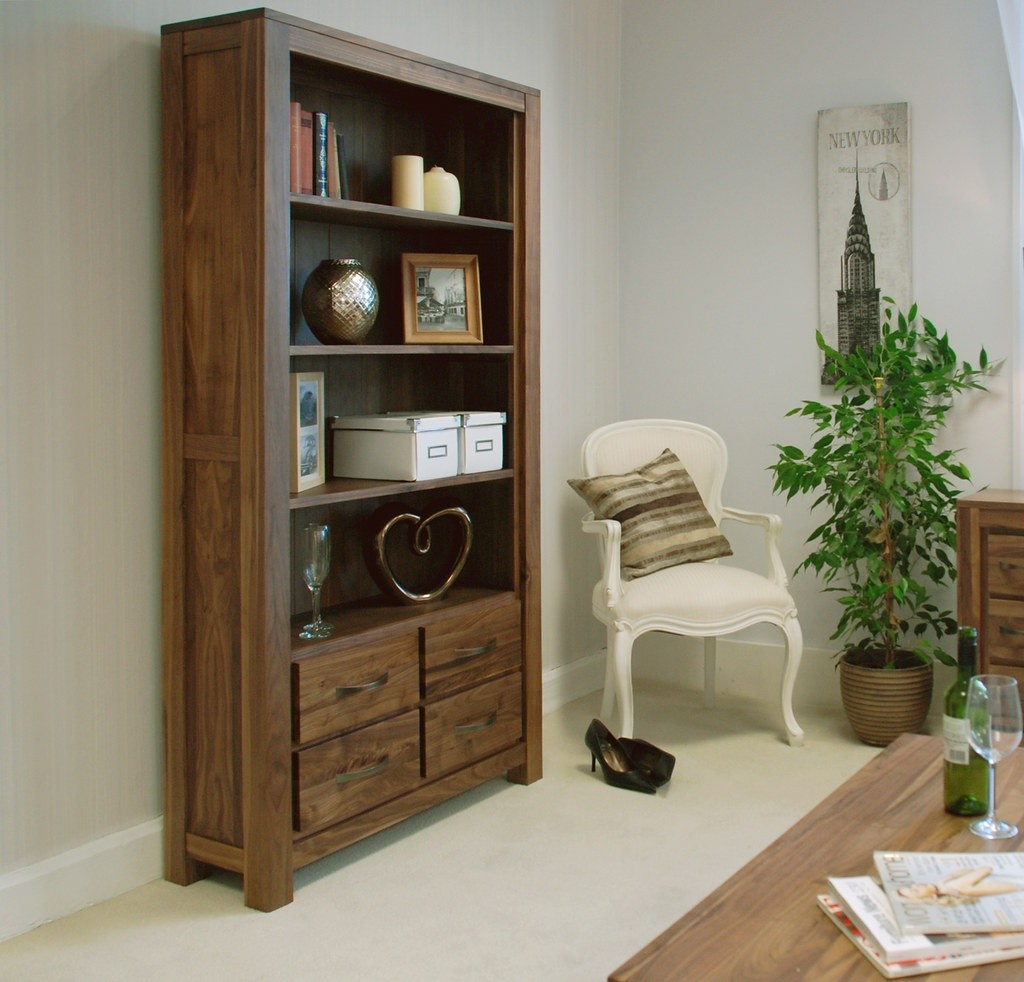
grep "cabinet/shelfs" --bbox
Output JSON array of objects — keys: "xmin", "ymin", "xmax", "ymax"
[
  {"xmin": 160, "ymin": 7, "xmax": 543, "ymax": 912},
  {"xmin": 955, "ymin": 485, "xmax": 1024, "ymax": 735}
]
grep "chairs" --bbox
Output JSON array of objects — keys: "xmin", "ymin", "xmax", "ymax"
[{"xmin": 580, "ymin": 416, "xmax": 810, "ymax": 744}]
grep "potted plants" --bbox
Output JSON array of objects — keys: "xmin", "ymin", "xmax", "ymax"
[{"xmin": 768, "ymin": 297, "xmax": 1001, "ymax": 750}]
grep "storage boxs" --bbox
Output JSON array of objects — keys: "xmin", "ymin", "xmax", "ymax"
[{"xmin": 329, "ymin": 408, "xmax": 507, "ymax": 482}]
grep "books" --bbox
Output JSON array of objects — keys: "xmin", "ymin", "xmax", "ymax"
[
  {"xmin": 290, "ymin": 101, "xmax": 354, "ymax": 200},
  {"xmin": 815, "ymin": 850, "xmax": 1024, "ymax": 980}
]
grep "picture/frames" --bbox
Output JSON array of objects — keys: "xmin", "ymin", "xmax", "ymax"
[
  {"xmin": 402, "ymin": 252, "xmax": 484, "ymax": 345},
  {"xmin": 291, "ymin": 369, "xmax": 326, "ymax": 493}
]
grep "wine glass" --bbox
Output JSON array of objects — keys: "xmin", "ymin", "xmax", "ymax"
[
  {"xmin": 298, "ymin": 521, "xmax": 334, "ymax": 640},
  {"xmin": 963, "ymin": 674, "xmax": 1024, "ymax": 839}
]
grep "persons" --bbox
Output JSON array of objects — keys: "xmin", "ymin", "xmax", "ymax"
[{"xmin": 897, "ymin": 867, "xmax": 1024, "ymax": 908}]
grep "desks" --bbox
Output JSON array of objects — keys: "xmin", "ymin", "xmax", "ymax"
[{"xmin": 609, "ymin": 733, "xmax": 1024, "ymax": 982}]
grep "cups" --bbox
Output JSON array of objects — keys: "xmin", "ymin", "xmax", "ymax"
[{"xmin": 391, "ymin": 155, "xmax": 425, "ymax": 212}]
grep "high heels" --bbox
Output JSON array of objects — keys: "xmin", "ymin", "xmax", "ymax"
[
  {"xmin": 585, "ymin": 719, "xmax": 657, "ymax": 793},
  {"xmin": 618, "ymin": 736, "xmax": 675, "ymax": 787}
]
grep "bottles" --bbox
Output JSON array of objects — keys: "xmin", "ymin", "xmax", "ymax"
[
  {"xmin": 302, "ymin": 258, "xmax": 379, "ymax": 345},
  {"xmin": 939, "ymin": 627, "xmax": 992, "ymax": 817},
  {"xmin": 423, "ymin": 166, "xmax": 463, "ymax": 215}
]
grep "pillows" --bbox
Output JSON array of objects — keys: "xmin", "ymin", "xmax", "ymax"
[{"xmin": 568, "ymin": 447, "xmax": 734, "ymax": 584}]
